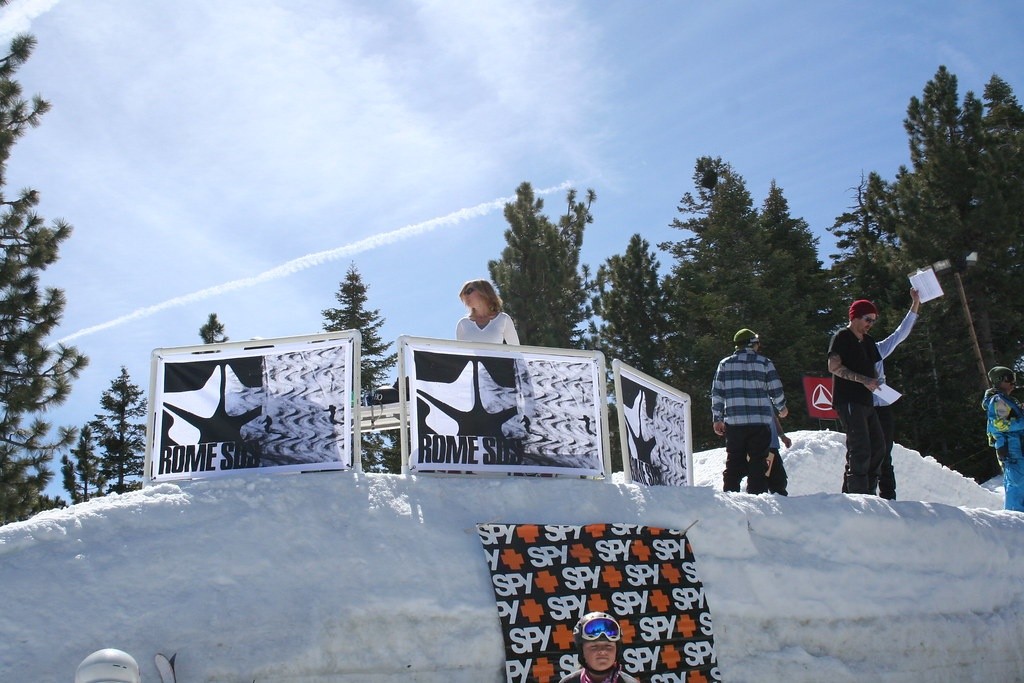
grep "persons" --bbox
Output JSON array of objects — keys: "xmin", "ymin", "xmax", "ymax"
[
  {"xmin": 982, "ymin": 367, "xmax": 1024, "ymax": 512},
  {"xmin": 842, "ymin": 287, "xmax": 919, "ymax": 501},
  {"xmin": 768, "ymin": 415, "xmax": 791, "ymax": 496},
  {"xmin": 711, "ymin": 329, "xmax": 788, "ymax": 495},
  {"xmin": 75, "ymin": 648, "xmax": 141, "ymax": 683},
  {"xmin": 456, "ymin": 281, "xmax": 520, "ymax": 345},
  {"xmin": 828, "ymin": 300, "xmax": 886, "ymax": 495},
  {"xmin": 559, "ymin": 611, "xmax": 640, "ymax": 683}
]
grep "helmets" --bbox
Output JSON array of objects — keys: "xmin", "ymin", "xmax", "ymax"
[
  {"xmin": 75, "ymin": 648, "xmax": 141, "ymax": 683},
  {"xmin": 572, "ymin": 611, "xmax": 621, "ymax": 652}
]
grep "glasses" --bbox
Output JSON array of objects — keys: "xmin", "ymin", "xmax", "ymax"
[
  {"xmin": 1004, "ymin": 374, "xmax": 1017, "ymax": 383},
  {"xmin": 463, "ymin": 287, "xmax": 477, "ymax": 295},
  {"xmin": 861, "ymin": 316, "xmax": 876, "ymax": 324},
  {"xmin": 754, "ymin": 338, "xmax": 760, "ymax": 346},
  {"xmin": 581, "ymin": 617, "xmax": 621, "ymax": 641}
]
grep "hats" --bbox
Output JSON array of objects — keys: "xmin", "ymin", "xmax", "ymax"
[
  {"xmin": 987, "ymin": 367, "xmax": 1013, "ymax": 389},
  {"xmin": 733, "ymin": 328, "xmax": 757, "ymax": 350},
  {"xmin": 849, "ymin": 300, "xmax": 878, "ymax": 321}
]
